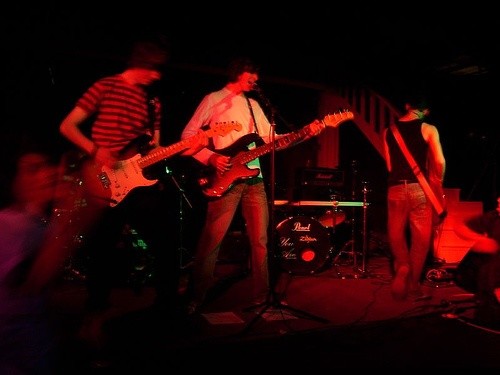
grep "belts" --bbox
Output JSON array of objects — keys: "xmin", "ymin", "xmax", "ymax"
[
  {"xmin": 238, "ymin": 177, "xmax": 263, "ymax": 185},
  {"xmin": 391, "ymin": 180, "xmax": 419, "ymax": 186}
]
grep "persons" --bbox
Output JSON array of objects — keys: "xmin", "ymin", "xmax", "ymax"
[
  {"xmin": 383, "ymin": 95, "xmax": 445, "ymax": 298},
  {"xmin": 0, "ymin": 146, "xmax": 109, "ymax": 375},
  {"xmin": 59, "ymin": 50, "xmax": 166, "ymax": 312},
  {"xmin": 451, "ymin": 196, "xmax": 500, "ymax": 330},
  {"xmin": 181, "ymin": 61, "xmax": 325, "ymax": 312}
]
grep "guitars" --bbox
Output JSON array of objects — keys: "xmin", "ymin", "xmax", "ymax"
[
  {"xmin": 191, "ymin": 107, "xmax": 356, "ymax": 201},
  {"xmin": 431, "ymin": 186, "xmax": 446, "ymax": 225},
  {"xmin": 80, "ymin": 120, "xmax": 244, "ymax": 210}
]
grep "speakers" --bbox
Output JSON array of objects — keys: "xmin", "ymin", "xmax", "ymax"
[{"xmin": 299, "ymin": 168, "xmax": 345, "ymax": 201}]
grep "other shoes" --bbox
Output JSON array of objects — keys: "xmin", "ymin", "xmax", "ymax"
[
  {"xmin": 392, "ymin": 263, "xmax": 410, "ymax": 296},
  {"xmin": 254, "ymin": 298, "xmax": 288, "ymax": 314},
  {"xmin": 408, "ymin": 281, "xmax": 422, "ymax": 294}
]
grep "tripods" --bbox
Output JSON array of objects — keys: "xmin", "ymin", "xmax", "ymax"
[
  {"xmin": 236, "ymin": 95, "xmax": 332, "ymax": 338},
  {"xmin": 331, "ymin": 160, "xmax": 364, "ymax": 277}
]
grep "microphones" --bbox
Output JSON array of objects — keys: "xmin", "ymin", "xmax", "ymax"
[{"xmin": 249, "ymin": 79, "xmax": 263, "ymax": 96}]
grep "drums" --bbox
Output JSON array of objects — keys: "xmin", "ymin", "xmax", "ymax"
[
  {"xmin": 269, "ymin": 214, "xmax": 332, "ymax": 277},
  {"xmin": 311, "ymin": 182, "xmax": 349, "ymax": 227}
]
